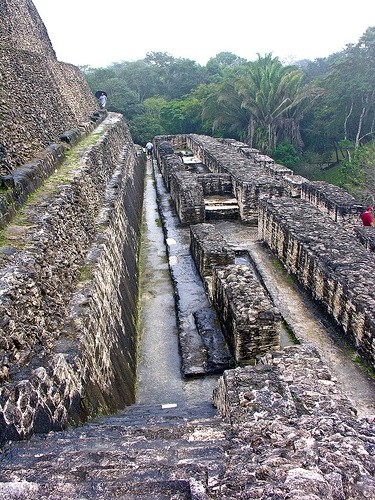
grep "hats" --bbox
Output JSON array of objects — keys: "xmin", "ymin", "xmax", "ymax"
[{"xmin": 367, "ymin": 207, "xmax": 373, "ymax": 210}]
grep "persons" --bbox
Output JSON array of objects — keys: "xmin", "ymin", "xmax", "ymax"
[
  {"xmin": 99, "ymin": 93, "xmax": 107, "ymax": 109},
  {"xmin": 361, "ymin": 207, "xmax": 374, "ymax": 228},
  {"xmin": 146, "ymin": 140, "xmax": 153, "ymax": 155}
]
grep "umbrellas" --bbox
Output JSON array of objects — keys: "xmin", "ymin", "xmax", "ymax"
[{"xmin": 94, "ymin": 91, "xmax": 107, "ymax": 99}]
